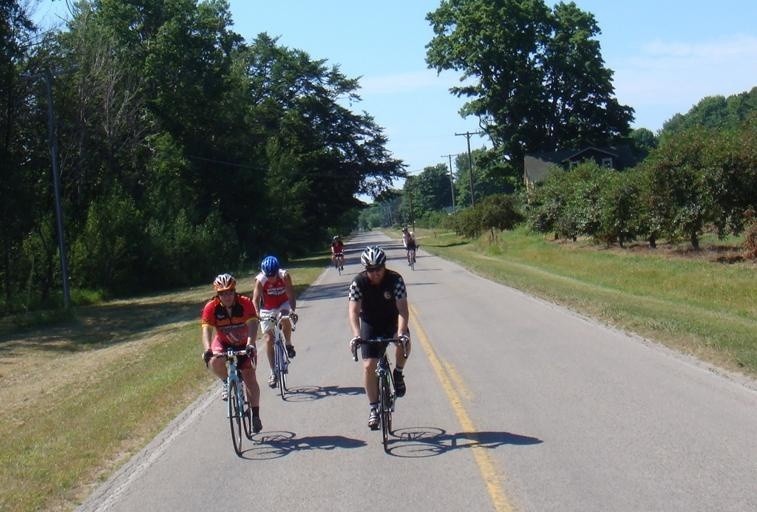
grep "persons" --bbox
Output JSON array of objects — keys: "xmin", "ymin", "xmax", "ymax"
[
  {"xmin": 329, "ymin": 234, "xmax": 344, "ymax": 271},
  {"xmin": 251, "ymin": 255, "xmax": 299, "ymax": 390},
  {"xmin": 346, "ymin": 244, "xmax": 412, "ymax": 432},
  {"xmin": 401, "ymin": 226, "xmax": 416, "ymax": 267},
  {"xmin": 200, "ymin": 272, "xmax": 264, "ymax": 435}
]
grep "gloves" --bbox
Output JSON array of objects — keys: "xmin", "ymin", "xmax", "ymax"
[
  {"xmin": 349, "ymin": 336, "xmax": 361, "ymax": 353},
  {"xmin": 202, "ymin": 349, "xmax": 213, "ymax": 363},
  {"xmin": 246, "ymin": 344, "xmax": 257, "ymax": 361},
  {"xmin": 289, "ymin": 311, "xmax": 298, "ymax": 325},
  {"xmin": 398, "ymin": 332, "xmax": 411, "ymax": 349}
]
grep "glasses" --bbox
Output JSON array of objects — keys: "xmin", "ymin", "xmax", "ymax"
[
  {"xmin": 365, "ymin": 265, "xmax": 385, "ymax": 272},
  {"xmin": 266, "ymin": 275, "xmax": 276, "ymax": 278},
  {"xmin": 218, "ymin": 290, "xmax": 235, "ymax": 296}
]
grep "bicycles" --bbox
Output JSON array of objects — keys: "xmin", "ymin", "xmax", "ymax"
[
  {"xmin": 403, "ymin": 245, "xmax": 420, "ymax": 270},
  {"xmin": 349, "ymin": 334, "xmax": 409, "ymax": 450},
  {"xmin": 256, "ymin": 312, "xmax": 298, "ymax": 400},
  {"xmin": 331, "ymin": 252, "xmax": 344, "ymax": 276},
  {"xmin": 202, "ymin": 343, "xmax": 259, "ymax": 455}
]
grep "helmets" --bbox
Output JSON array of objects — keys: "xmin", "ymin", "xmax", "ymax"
[
  {"xmin": 213, "ymin": 273, "xmax": 236, "ymax": 291},
  {"xmin": 333, "ymin": 236, "xmax": 340, "ymax": 241},
  {"xmin": 261, "ymin": 256, "xmax": 279, "ymax": 276},
  {"xmin": 401, "ymin": 226, "xmax": 408, "ymax": 232},
  {"xmin": 361, "ymin": 245, "xmax": 387, "ymax": 268}
]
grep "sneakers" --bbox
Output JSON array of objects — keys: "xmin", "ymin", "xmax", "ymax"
[
  {"xmin": 222, "ymin": 383, "xmax": 228, "ymax": 401},
  {"xmin": 252, "ymin": 416, "xmax": 263, "ymax": 433},
  {"xmin": 369, "ymin": 407, "xmax": 380, "ymax": 428},
  {"xmin": 286, "ymin": 345, "xmax": 296, "ymax": 358},
  {"xmin": 393, "ymin": 368, "xmax": 406, "ymax": 397},
  {"xmin": 268, "ymin": 374, "xmax": 278, "ymax": 388}
]
